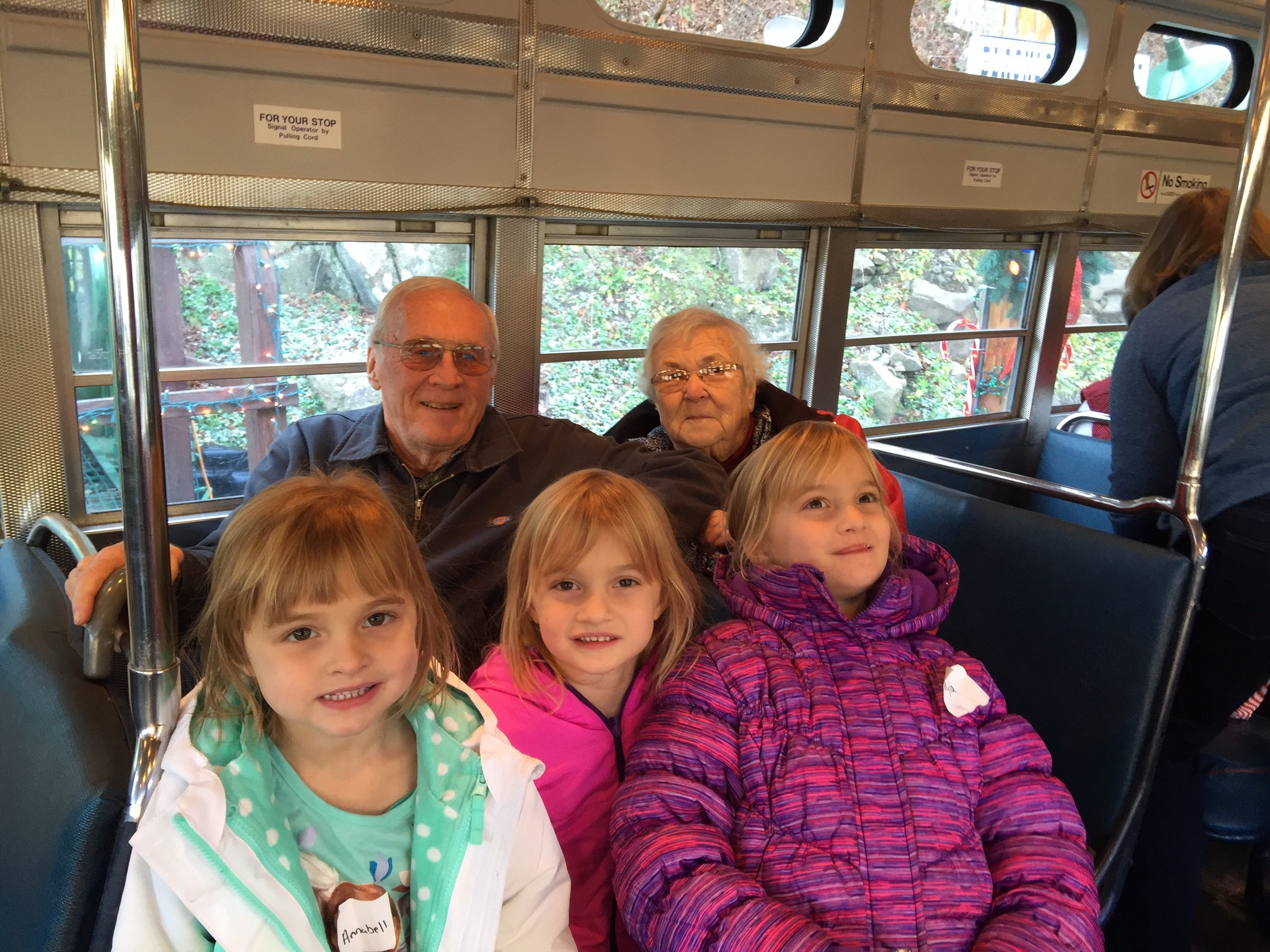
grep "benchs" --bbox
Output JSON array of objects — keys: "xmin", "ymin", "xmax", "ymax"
[
  {"xmin": 4, "ymin": 511, "xmax": 158, "ymax": 946},
  {"xmin": 864, "ymin": 441, "xmax": 1213, "ymax": 925}
]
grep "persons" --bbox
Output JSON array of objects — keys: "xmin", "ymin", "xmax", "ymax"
[
  {"xmin": 97, "ymin": 464, "xmax": 575, "ymax": 952},
  {"xmin": 610, "ymin": 418, "xmax": 1109, "ymax": 952},
  {"xmin": 1104, "ymin": 181, "xmax": 1270, "ymax": 952},
  {"xmin": 459, "ymin": 463, "xmax": 701, "ymax": 951},
  {"xmin": 62, "ymin": 276, "xmax": 736, "ymax": 675},
  {"xmin": 606, "ymin": 302, "xmax": 812, "ymax": 490}
]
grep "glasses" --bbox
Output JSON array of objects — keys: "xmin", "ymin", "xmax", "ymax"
[
  {"xmin": 651, "ymin": 361, "xmax": 744, "ymax": 393},
  {"xmin": 374, "ymin": 338, "xmax": 499, "ymax": 376}
]
